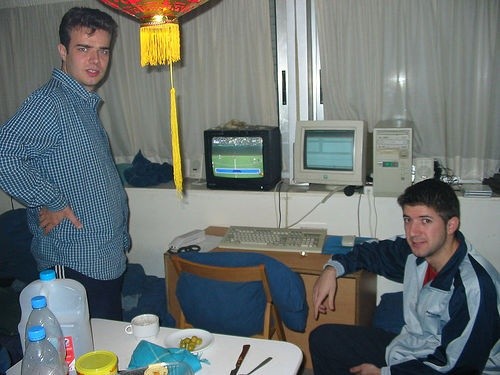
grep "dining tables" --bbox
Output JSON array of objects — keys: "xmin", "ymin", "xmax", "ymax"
[{"xmin": 4, "ymin": 318, "xmax": 304, "ymax": 375}]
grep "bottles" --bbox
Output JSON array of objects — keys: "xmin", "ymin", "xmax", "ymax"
[
  {"xmin": 26, "ymin": 296, "xmax": 64, "ymax": 368},
  {"xmin": 22, "ymin": 326, "xmax": 65, "ymax": 375},
  {"xmin": 19, "ymin": 269, "xmax": 95, "ymax": 375}
]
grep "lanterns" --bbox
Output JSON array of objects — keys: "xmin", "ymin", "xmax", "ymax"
[{"xmin": 98, "ymin": 0, "xmax": 211, "ymax": 192}]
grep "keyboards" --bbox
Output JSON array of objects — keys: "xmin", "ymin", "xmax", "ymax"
[{"xmin": 218, "ymin": 226, "xmax": 327, "ymax": 254}]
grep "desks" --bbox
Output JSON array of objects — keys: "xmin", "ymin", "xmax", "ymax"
[
  {"xmin": 164, "ymin": 226, "xmax": 377, "ymax": 369},
  {"xmin": 123, "ymin": 178, "xmax": 500, "ymax": 278}
]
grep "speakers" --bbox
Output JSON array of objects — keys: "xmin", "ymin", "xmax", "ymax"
[{"xmin": 415, "ymin": 158, "xmax": 435, "ymax": 184}]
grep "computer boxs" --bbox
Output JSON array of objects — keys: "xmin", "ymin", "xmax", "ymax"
[{"xmin": 373, "ymin": 128, "xmax": 413, "ymax": 198}]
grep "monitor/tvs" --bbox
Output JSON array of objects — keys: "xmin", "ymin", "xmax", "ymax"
[
  {"xmin": 294, "ymin": 120, "xmax": 368, "ymax": 190},
  {"xmin": 204, "ymin": 126, "xmax": 282, "ymax": 191}
]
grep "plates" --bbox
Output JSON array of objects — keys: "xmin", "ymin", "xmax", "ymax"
[{"xmin": 165, "ymin": 328, "xmax": 213, "ymax": 352}]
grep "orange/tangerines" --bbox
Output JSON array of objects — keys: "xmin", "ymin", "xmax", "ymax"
[{"xmin": 180, "ymin": 336, "xmax": 202, "ymax": 350}]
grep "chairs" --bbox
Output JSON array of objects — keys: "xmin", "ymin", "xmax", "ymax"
[{"xmin": 169, "ymin": 255, "xmax": 287, "ymax": 342}]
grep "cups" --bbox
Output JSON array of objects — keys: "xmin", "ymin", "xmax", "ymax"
[{"xmin": 125, "ymin": 314, "xmax": 159, "ymax": 342}]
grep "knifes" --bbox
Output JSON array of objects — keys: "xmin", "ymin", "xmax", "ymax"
[{"xmin": 230, "ymin": 345, "xmax": 250, "ymax": 375}]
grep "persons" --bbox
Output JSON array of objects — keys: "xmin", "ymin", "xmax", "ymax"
[
  {"xmin": 0, "ymin": 7, "xmax": 132, "ymax": 322},
  {"xmin": 308, "ymin": 179, "xmax": 500, "ymax": 375}
]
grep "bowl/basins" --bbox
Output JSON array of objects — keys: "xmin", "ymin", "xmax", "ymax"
[{"xmin": 119, "ymin": 362, "xmax": 196, "ymax": 375}]
